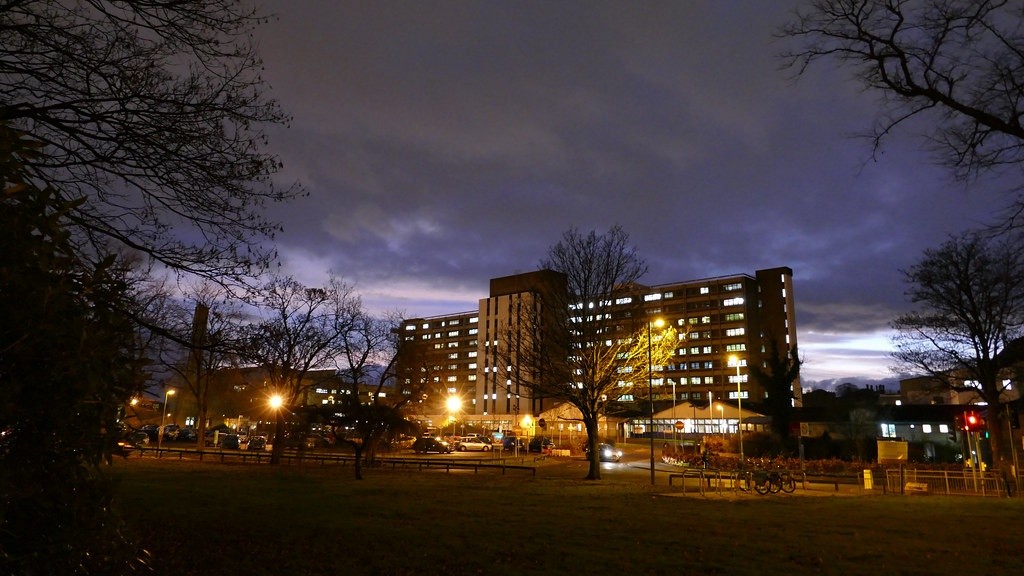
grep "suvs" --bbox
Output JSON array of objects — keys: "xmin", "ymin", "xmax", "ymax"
[{"xmin": 528, "ymin": 438, "xmax": 556, "ymax": 455}]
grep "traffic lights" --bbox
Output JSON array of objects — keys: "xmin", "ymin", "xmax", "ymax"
[{"xmin": 968, "ymin": 414, "xmax": 977, "ymax": 430}]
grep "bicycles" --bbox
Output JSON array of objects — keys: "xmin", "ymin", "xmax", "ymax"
[{"xmin": 743, "ymin": 459, "xmax": 796, "ymax": 495}]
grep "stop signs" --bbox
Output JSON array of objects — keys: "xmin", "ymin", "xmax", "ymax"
[
  {"xmin": 538, "ymin": 418, "xmax": 546, "ymax": 427},
  {"xmin": 675, "ymin": 421, "xmax": 685, "ymax": 430}
]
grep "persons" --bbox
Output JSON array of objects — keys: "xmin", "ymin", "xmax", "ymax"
[{"xmin": 1000, "ymin": 456, "xmax": 1014, "ymax": 497}]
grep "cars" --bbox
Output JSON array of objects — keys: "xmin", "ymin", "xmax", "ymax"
[
  {"xmin": 502, "ymin": 436, "xmax": 525, "ymax": 452},
  {"xmin": 585, "ymin": 443, "xmax": 622, "ymax": 463},
  {"xmin": 412, "ymin": 437, "xmax": 453, "ymax": 456},
  {"xmin": 390, "ymin": 435, "xmax": 417, "ymax": 450},
  {"xmin": 111, "ymin": 419, "xmax": 365, "ymax": 453},
  {"xmin": 453, "ymin": 433, "xmax": 493, "ymax": 453}
]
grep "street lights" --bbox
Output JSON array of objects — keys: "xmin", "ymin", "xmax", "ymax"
[
  {"xmin": 155, "ymin": 390, "xmax": 176, "ymax": 458},
  {"xmin": 729, "ymin": 353, "xmax": 744, "ymax": 461},
  {"xmin": 646, "ymin": 318, "xmax": 665, "ymax": 485},
  {"xmin": 448, "ymin": 395, "xmax": 460, "ymax": 437},
  {"xmin": 523, "ymin": 416, "xmax": 533, "ymax": 456},
  {"xmin": 267, "ymin": 395, "xmax": 282, "ymax": 424}
]
